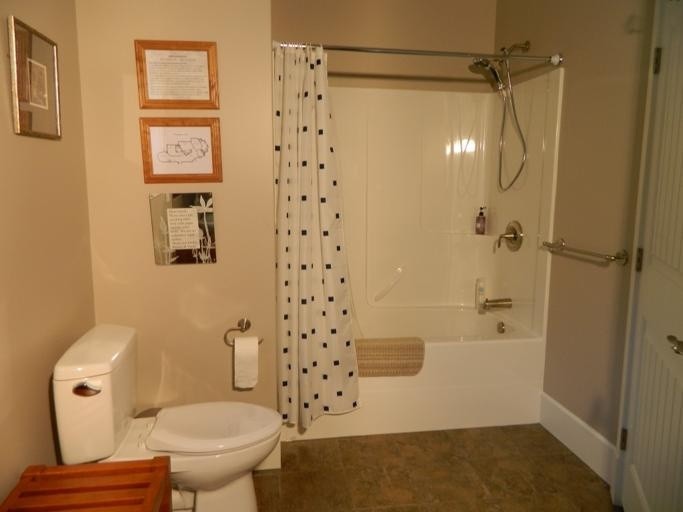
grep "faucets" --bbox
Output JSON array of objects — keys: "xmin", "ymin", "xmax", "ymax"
[{"xmin": 480, "ymin": 297, "xmax": 513, "ymax": 314}]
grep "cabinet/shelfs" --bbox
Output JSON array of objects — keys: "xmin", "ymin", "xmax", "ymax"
[{"xmin": 0, "ymin": 454, "xmax": 177, "ymax": 512}]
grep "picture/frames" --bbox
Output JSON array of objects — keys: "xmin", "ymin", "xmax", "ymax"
[
  {"xmin": 146, "ymin": 192, "xmax": 217, "ymax": 266},
  {"xmin": 137, "ymin": 116, "xmax": 223, "ymax": 184},
  {"xmin": 8, "ymin": 14, "xmax": 63, "ymax": 140},
  {"xmin": 132, "ymin": 39, "xmax": 220, "ymax": 112}
]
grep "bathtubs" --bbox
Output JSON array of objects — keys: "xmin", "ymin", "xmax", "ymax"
[{"xmin": 281, "ymin": 304, "xmax": 545, "ymax": 444}]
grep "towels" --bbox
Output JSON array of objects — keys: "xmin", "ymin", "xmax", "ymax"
[{"xmin": 351, "ymin": 336, "xmax": 426, "ymax": 378}]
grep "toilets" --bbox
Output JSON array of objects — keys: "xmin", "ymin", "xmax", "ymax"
[{"xmin": 51, "ymin": 322, "xmax": 284, "ymax": 511}]
